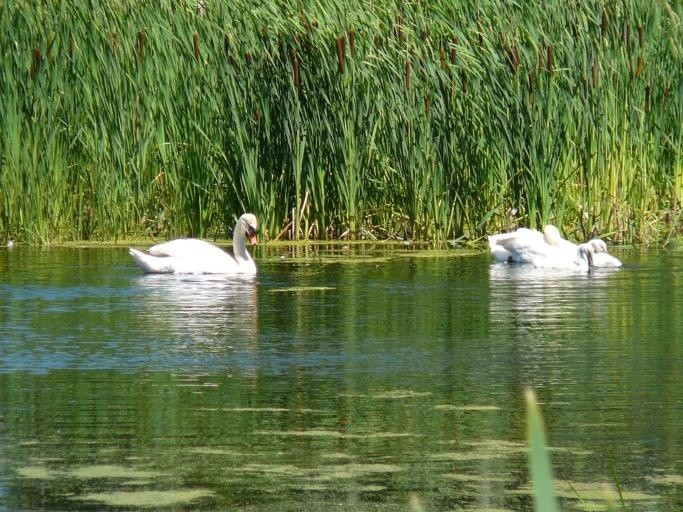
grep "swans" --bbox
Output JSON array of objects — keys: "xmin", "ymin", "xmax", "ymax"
[
  {"xmin": 128, "ymin": 213, "xmax": 258, "ymax": 276},
  {"xmin": 487, "ymin": 225, "xmax": 622, "ymax": 273}
]
